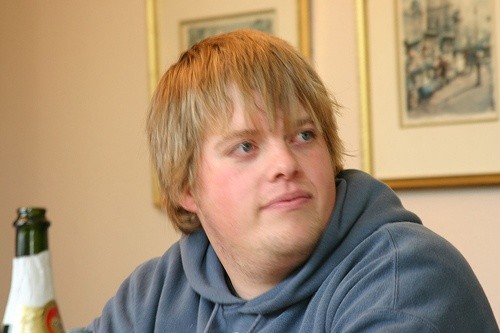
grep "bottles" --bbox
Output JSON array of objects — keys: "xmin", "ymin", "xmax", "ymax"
[{"xmin": 2, "ymin": 205, "xmax": 63, "ymax": 333}]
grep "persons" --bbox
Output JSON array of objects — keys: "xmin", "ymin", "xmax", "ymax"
[{"xmin": 67, "ymin": 29, "xmax": 499, "ymax": 333}]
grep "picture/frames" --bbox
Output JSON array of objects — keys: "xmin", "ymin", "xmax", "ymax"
[
  {"xmin": 355, "ymin": 1, "xmax": 500, "ymax": 191},
  {"xmin": 145, "ymin": 1, "xmax": 314, "ymax": 102}
]
